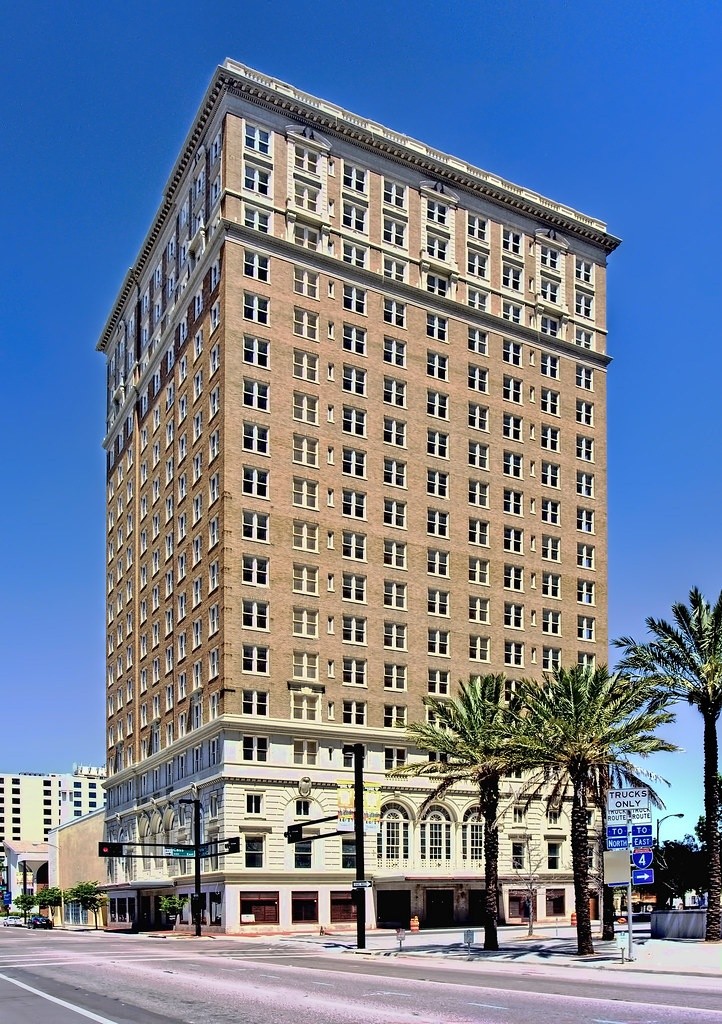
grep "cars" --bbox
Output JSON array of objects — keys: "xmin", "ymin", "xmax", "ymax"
[
  {"xmin": 28, "ymin": 917, "xmax": 52, "ymax": 929},
  {"xmin": 3, "ymin": 916, "xmax": 22, "ymax": 927}
]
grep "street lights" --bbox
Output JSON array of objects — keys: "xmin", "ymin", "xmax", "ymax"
[
  {"xmin": 343, "ymin": 743, "xmax": 366, "ymax": 950},
  {"xmin": 21, "ymin": 860, "xmax": 26, "ymax": 924},
  {"xmin": 178, "ymin": 798, "xmax": 201, "ymax": 936},
  {"xmin": 656, "ymin": 814, "xmax": 684, "ymax": 850},
  {"xmin": 32, "ymin": 842, "xmax": 65, "ymax": 927},
  {"xmin": 0, "ymin": 866, "xmax": 9, "ymax": 915}
]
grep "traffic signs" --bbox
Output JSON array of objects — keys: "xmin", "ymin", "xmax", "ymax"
[{"xmin": 352, "ymin": 880, "xmax": 374, "ymax": 887}]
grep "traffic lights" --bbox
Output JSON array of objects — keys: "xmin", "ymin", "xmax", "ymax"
[
  {"xmin": 99, "ymin": 842, "xmax": 123, "ymax": 857},
  {"xmin": 284, "ymin": 824, "xmax": 303, "ymax": 844}
]
